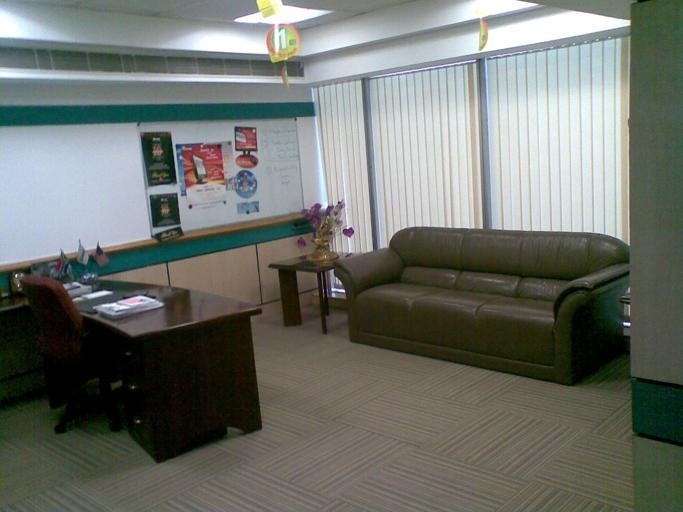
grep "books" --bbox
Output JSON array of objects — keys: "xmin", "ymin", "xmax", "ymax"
[{"xmin": 63, "ymin": 280, "xmax": 165, "ymax": 320}]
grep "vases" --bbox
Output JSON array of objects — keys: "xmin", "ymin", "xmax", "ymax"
[{"xmin": 306, "ymin": 238, "xmax": 339, "ymax": 265}]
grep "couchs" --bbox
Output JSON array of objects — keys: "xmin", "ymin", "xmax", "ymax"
[{"xmin": 334, "ymin": 222, "xmax": 631, "ymax": 387}]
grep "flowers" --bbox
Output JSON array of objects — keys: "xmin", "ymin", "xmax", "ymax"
[{"xmin": 295, "ymin": 200, "xmax": 355, "ymax": 251}]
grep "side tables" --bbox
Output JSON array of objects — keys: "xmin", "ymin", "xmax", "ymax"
[{"xmin": 266, "ymin": 249, "xmax": 359, "ymax": 334}]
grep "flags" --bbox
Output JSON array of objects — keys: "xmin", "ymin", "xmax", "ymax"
[
  {"xmin": 76, "ymin": 242, "xmax": 90, "ymax": 265},
  {"xmin": 94, "ymin": 244, "xmax": 109, "ymax": 267},
  {"xmin": 57, "ymin": 250, "xmax": 70, "ymax": 274}
]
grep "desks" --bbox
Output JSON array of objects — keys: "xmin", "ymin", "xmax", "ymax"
[{"xmin": 0, "ymin": 276, "xmax": 263, "ymax": 464}]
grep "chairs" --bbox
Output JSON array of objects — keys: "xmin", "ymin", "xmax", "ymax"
[{"xmin": 18, "ymin": 275, "xmax": 137, "ymax": 435}]
